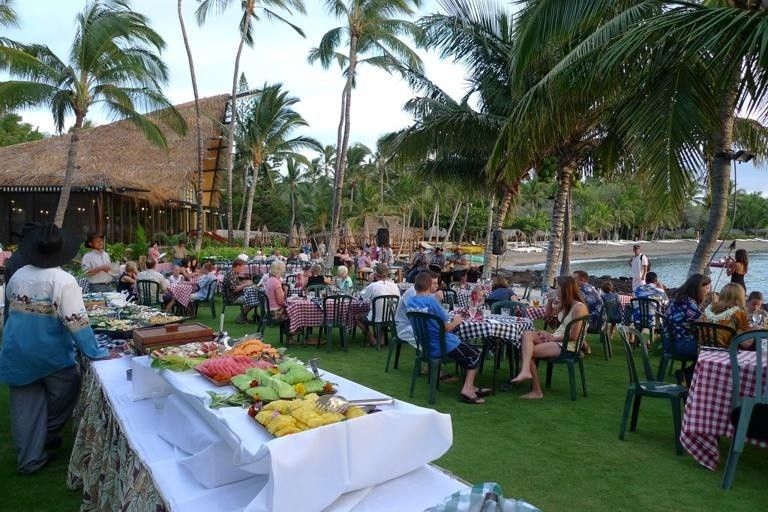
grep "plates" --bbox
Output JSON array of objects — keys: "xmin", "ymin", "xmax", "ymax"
[{"xmin": 197, "ymin": 359, "xmax": 232, "ymax": 386}]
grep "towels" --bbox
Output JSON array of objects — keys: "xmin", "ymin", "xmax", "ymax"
[
  {"xmin": 452, "ymin": 304, "xmax": 471, "ymax": 320},
  {"xmin": 312, "ymin": 296, "xmax": 325, "ymax": 312}
]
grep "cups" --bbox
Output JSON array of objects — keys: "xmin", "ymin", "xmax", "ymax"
[
  {"xmin": 483, "ymin": 310, "xmax": 491, "ymax": 320},
  {"xmin": 501, "ymin": 308, "xmax": 510, "ymax": 319},
  {"xmin": 753, "ymin": 313, "xmax": 762, "ymax": 324},
  {"xmin": 469, "ymin": 308, "xmax": 477, "ymax": 319}
]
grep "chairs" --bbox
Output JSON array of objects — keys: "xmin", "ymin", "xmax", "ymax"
[
  {"xmin": 307, "ymin": 285, "xmax": 330, "ymax": 298},
  {"xmin": 588, "ymin": 302, "xmax": 613, "ymax": 361},
  {"xmin": 692, "ymin": 321, "xmax": 738, "ymax": 349},
  {"xmin": 137, "ymin": 279, "xmax": 165, "ymax": 309},
  {"xmin": 438, "ymin": 287, "xmax": 459, "ymax": 305},
  {"xmin": 616, "ymin": 325, "xmax": 689, "ymax": 456},
  {"xmin": 534, "ymin": 314, "xmax": 593, "ymax": 402},
  {"xmin": 630, "ymin": 297, "xmax": 661, "ymax": 351},
  {"xmin": 405, "ymin": 311, "xmax": 466, "ymax": 405},
  {"xmin": 286, "ymin": 274, "xmax": 298, "ymax": 283},
  {"xmin": 384, "ymin": 299, "xmax": 419, "ymax": 372},
  {"xmin": 193, "ymin": 279, "xmax": 218, "ymax": 320},
  {"xmin": 721, "ymin": 328, "xmax": 768, "ymax": 491},
  {"xmin": 654, "ymin": 305, "xmax": 696, "ymax": 383},
  {"xmin": 364, "ymin": 294, "xmax": 400, "ymax": 351},
  {"xmin": 491, "ymin": 301, "xmax": 527, "ymax": 317},
  {"xmin": 257, "ymin": 290, "xmax": 286, "ymax": 343},
  {"xmin": 316, "ymin": 294, "xmax": 353, "ymax": 354}
]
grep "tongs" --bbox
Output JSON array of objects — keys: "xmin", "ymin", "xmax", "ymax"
[{"xmin": 315, "ymin": 395, "xmax": 394, "ymax": 414}]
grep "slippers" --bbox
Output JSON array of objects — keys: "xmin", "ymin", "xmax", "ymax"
[
  {"xmin": 439, "ymin": 374, "xmax": 460, "ymax": 383},
  {"xmin": 475, "ymin": 388, "xmax": 492, "ymax": 396},
  {"xmin": 459, "ymin": 395, "xmax": 485, "ymax": 404}
]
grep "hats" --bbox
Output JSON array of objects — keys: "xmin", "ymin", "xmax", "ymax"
[
  {"xmin": 17, "ymin": 221, "xmax": 82, "ymax": 269},
  {"xmin": 435, "ymin": 246, "xmax": 444, "ymax": 252},
  {"xmin": 83, "ymin": 229, "xmax": 104, "ymax": 249},
  {"xmin": 454, "ymin": 246, "xmax": 464, "ymax": 251},
  {"xmin": 415, "ymin": 243, "xmax": 426, "ymax": 250}
]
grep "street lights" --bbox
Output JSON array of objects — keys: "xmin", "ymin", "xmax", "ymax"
[{"xmin": 704, "ymin": 147, "xmax": 756, "ymax": 272}]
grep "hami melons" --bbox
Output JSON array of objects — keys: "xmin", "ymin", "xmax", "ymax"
[{"xmin": 225, "ymin": 338, "xmax": 280, "ymax": 356}]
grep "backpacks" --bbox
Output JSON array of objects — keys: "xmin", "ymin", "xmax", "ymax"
[{"xmin": 632, "ymin": 255, "xmax": 649, "ymax": 272}]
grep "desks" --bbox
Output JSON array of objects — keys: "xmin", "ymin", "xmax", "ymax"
[{"xmin": 64, "ymin": 336, "xmax": 542, "ymax": 511}]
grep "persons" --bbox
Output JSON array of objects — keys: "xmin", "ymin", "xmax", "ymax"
[
  {"xmin": 699, "ymin": 283, "xmax": 754, "ymax": 347},
  {"xmin": 254, "ymin": 248, "xmax": 310, "ymax": 261},
  {"xmin": 334, "ymin": 241, "xmax": 394, "ymax": 272},
  {"xmin": 663, "ymin": 273, "xmax": 711, "ymax": 390},
  {"xmin": 510, "ymin": 275, "xmax": 590, "ymax": 399},
  {"xmin": 135, "ymin": 259, "xmax": 176, "ymax": 312},
  {"xmin": 727, "ymin": 249, "xmax": 749, "ymax": 291},
  {"xmin": 201, "ymin": 259, "xmax": 215, "ymax": 275},
  {"xmin": 335, "ymin": 265, "xmax": 353, "ymax": 294},
  {"xmin": 394, "ymin": 270, "xmax": 463, "ymax": 383},
  {"xmin": 572, "ymin": 270, "xmax": 603, "ymax": 332},
  {"xmin": 449, "ymin": 246, "xmax": 470, "ymax": 283},
  {"xmin": 190, "ymin": 254, "xmax": 198, "ymax": 273},
  {"xmin": 629, "ymin": 272, "xmax": 669, "ymax": 348},
  {"xmin": 453, "ymin": 277, "xmax": 475, "ymax": 294},
  {"xmin": 172, "ymin": 240, "xmax": 186, "ymax": 264},
  {"xmin": 0, "ymin": 223, "xmax": 122, "ymax": 475},
  {"xmin": 601, "ymin": 281, "xmax": 623, "ymax": 341},
  {"xmin": 319, "ymin": 240, "xmax": 325, "ymax": 255},
  {"xmin": 148, "ymin": 240, "xmax": 161, "ymax": 264},
  {"xmin": 429, "ymin": 247, "xmax": 445, "ymax": 282},
  {"xmin": 138, "ymin": 256, "xmax": 148, "ymax": 272},
  {"xmin": 263, "ymin": 260, "xmax": 300, "ymax": 345},
  {"xmin": 221, "ymin": 258, "xmax": 256, "ymax": 324},
  {"xmin": 629, "ymin": 245, "xmax": 648, "ymax": 295},
  {"xmin": 487, "ymin": 279, "xmax": 519, "ymax": 316},
  {"xmin": 406, "ymin": 243, "xmax": 428, "ymax": 283},
  {"xmin": 746, "ymin": 291, "xmax": 768, "ymax": 326},
  {"xmin": 407, "ymin": 272, "xmax": 493, "ymax": 404},
  {"xmin": 81, "ymin": 233, "xmax": 114, "ymax": 293},
  {"xmin": 354, "ymin": 264, "xmax": 400, "ymax": 348},
  {"xmin": 179, "ymin": 258, "xmax": 198, "ymax": 278},
  {"xmin": 307, "ymin": 239, "xmax": 311, "ymax": 249},
  {"xmin": 302, "ymin": 265, "xmax": 331, "ymax": 292},
  {"xmin": 118, "ymin": 263, "xmax": 138, "ymax": 300},
  {"xmin": 187, "ymin": 263, "xmax": 217, "ymax": 316}
]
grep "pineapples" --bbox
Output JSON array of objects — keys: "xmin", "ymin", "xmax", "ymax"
[{"xmin": 255, "ymin": 393, "xmax": 363, "ymax": 438}]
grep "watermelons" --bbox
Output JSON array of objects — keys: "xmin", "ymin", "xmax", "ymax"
[{"xmin": 198, "ymin": 354, "xmax": 257, "ymax": 378}]
